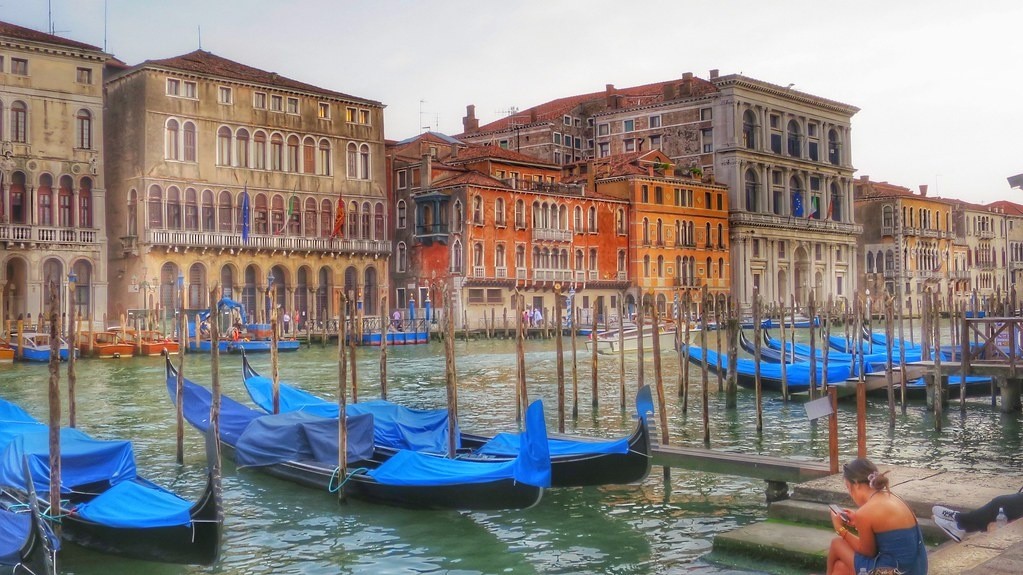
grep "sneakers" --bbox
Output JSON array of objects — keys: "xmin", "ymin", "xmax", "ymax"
[
  {"xmin": 931, "ymin": 505, "xmax": 961, "ymax": 521},
  {"xmin": 931, "ymin": 514, "xmax": 966, "ymax": 543}
]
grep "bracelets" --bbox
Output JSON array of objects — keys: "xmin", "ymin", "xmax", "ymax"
[
  {"xmin": 835, "ymin": 527, "xmax": 845, "ymax": 536},
  {"xmin": 843, "ymin": 530, "xmax": 848, "ymax": 540}
]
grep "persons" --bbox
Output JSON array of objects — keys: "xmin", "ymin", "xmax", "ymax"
[
  {"xmin": 522, "ymin": 304, "xmax": 542, "ymax": 328},
  {"xmin": 200, "ymin": 322, "xmax": 211, "ymax": 334},
  {"xmin": 233, "ymin": 318, "xmax": 241, "ymax": 332},
  {"xmin": 295, "ymin": 310, "xmax": 303, "ymax": 332},
  {"xmin": 284, "ymin": 312, "xmax": 290, "ymax": 333},
  {"xmin": 931, "ymin": 485, "xmax": 1023, "ymax": 543},
  {"xmin": 826, "ymin": 457, "xmax": 928, "ymax": 575},
  {"xmin": 393, "ymin": 309, "xmax": 400, "ymax": 328}
]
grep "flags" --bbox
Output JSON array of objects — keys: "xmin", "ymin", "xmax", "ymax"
[
  {"xmin": 808, "ymin": 191, "xmax": 817, "ymax": 218},
  {"xmin": 288, "ymin": 185, "xmax": 296, "ymax": 215},
  {"xmin": 243, "ymin": 185, "xmax": 249, "ymax": 243},
  {"xmin": 329, "ymin": 192, "xmax": 344, "ymax": 240},
  {"xmin": 828, "ymin": 200, "xmax": 833, "ymax": 221},
  {"xmin": 792, "ymin": 190, "xmax": 803, "ymax": 217}
]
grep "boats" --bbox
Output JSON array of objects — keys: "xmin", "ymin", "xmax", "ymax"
[
  {"xmin": 0, "ymin": 487, "xmax": 55, "ymax": 575},
  {"xmin": 239, "ymin": 346, "xmax": 658, "ymax": 488},
  {"xmin": 772, "ymin": 316, "xmax": 819, "ymax": 328},
  {"xmin": 674, "ymin": 328, "xmax": 998, "ymax": 391},
  {"xmin": 697, "ymin": 317, "xmax": 772, "ymax": 329},
  {"xmin": 584, "ymin": 324, "xmax": 702, "ymax": 355},
  {"xmin": 105, "ymin": 329, "xmax": 179, "ymax": 356},
  {"xmin": 3, "ymin": 333, "xmax": 79, "ymax": 361},
  {"xmin": 65, "ymin": 331, "xmax": 133, "ymax": 359},
  {"xmin": 859, "ymin": 325, "xmax": 1021, "ymax": 361},
  {"xmin": 0, "ymin": 396, "xmax": 224, "ymax": 563},
  {"xmin": 162, "ymin": 350, "xmax": 552, "ymax": 510},
  {"xmin": 819, "ymin": 329, "xmax": 948, "ymax": 362},
  {"xmin": 0, "ymin": 336, "xmax": 15, "ymax": 363},
  {"xmin": 763, "ymin": 328, "xmax": 936, "ymax": 365}
]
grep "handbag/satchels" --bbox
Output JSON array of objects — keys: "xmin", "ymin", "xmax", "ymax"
[{"xmin": 867, "ymin": 552, "xmax": 907, "ymax": 575}]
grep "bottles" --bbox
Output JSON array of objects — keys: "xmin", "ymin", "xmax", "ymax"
[
  {"xmin": 858, "ymin": 567, "xmax": 869, "ymax": 575},
  {"xmin": 996, "ymin": 508, "xmax": 1007, "ymax": 542}
]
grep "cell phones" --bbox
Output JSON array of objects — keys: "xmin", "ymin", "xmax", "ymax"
[{"xmin": 829, "ymin": 505, "xmax": 849, "ymax": 521}]
